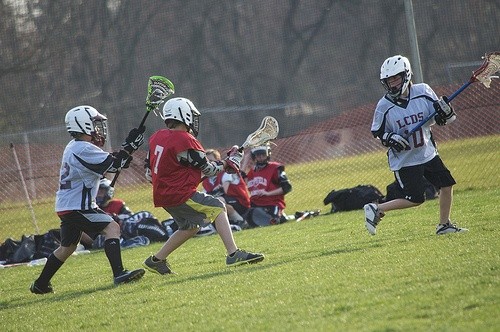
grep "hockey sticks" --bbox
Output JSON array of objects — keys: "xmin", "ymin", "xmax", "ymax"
[
  {"xmin": 403, "ymin": 51, "xmax": 500, "ymax": 141},
  {"xmin": 98, "ymin": 75, "xmax": 175, "ymax": 210},
  {"xmin": 201, "ymin": 115, "xmax": 280, "ymax": 184}
]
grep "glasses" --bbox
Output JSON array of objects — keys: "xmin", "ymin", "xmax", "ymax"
[{"xmin": 254, "ymin": 151, "xmax": 266, "ymax": 155}]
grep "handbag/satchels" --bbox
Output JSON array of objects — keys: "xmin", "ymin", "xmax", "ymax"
[
  {"xmin": 0, "ymin": 228, "xmax": 60, "ymax": 265},
  {"xmin": 137, "ymin": 217, "xmax": 169, "ymax": 243},
  {"xmin": 386, "ymin": 176, "xmax": 440, "ymax": 202},
  {"xmin": 324, "ymin": 185, "xmax": 384, "ymax": 214}
]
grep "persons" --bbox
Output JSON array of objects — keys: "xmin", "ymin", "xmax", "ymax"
[
  {"xmin": 77, "ymin": 178, "xmax": 136, "ymax": 251},
  {"xmin": 202, "ymin": 149, "xmax": 251, "ymax": 232},
  {"xmin": 245, "ymin": 142, "xmax": 292, "ymax": 227},
  {"xmin": 363, "ymin": 56, "xmax": 469, "ymax": 235},
  {"xmin": 143, "ymin": 98, "xmax": 265, "ymax": 278},
  {"xmin": 30, "ymin": 105, "xmax": 145, "ymax": 294}
]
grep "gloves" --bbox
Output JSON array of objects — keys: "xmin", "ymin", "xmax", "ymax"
[
  {"xmin": 121, "ymin": 128, "xmax": 144, "ymax": 154},
  {"xmin": 433, "ymin": 96, "xmax": 457, "ymax": 122},
  {"xmin": 225, "ymin": 145, "xmax": 244, "ymax": 174},
  {"xmin": 382, "ymin": 132, "xmax": 411, "ymax": 153},
  {"xmin": 112, "ymin": 152, "xmax": 133, "ymax": 169}
]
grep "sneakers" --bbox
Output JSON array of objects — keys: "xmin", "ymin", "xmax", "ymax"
[
  {"xmin": 436, "ymin": 219, "xmax": 469, "ymax": 235},
  {"xmin": 30, "ymin": 280, "xmax": 53, "ymax": 294},
  {"xmin": 363, "ymin": 203, "xmax": 385, "ymax": 235},
  {"xmin": 115, "ymin": 269, "xmax": 145, "ymax": 285},
  {"xmin": 226, "ymin": 248, "xmax": 264, "ymax": 267},
  {"xmin": 142, "ymin": 255, "xmax": 178, "ymax": 275}
]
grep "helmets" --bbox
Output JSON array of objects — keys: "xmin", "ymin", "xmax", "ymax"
[
  {"xmin": 250, "ymin": 143, "xmax": 272, "ymax": 161},
  {"xmin": 65, "ymin": 106, "xmax": 108, "ymax": 136},
  {"xmin": 380, "ymin": 55, "xmax": 411, "ymax": 97},
  {"xmin": 163, "ymin": 97, "xmax": 201, "ymax": 130},
  {"xmin": 99, "ymin": 178, "xmax": 114, "ymax": 199}
]
